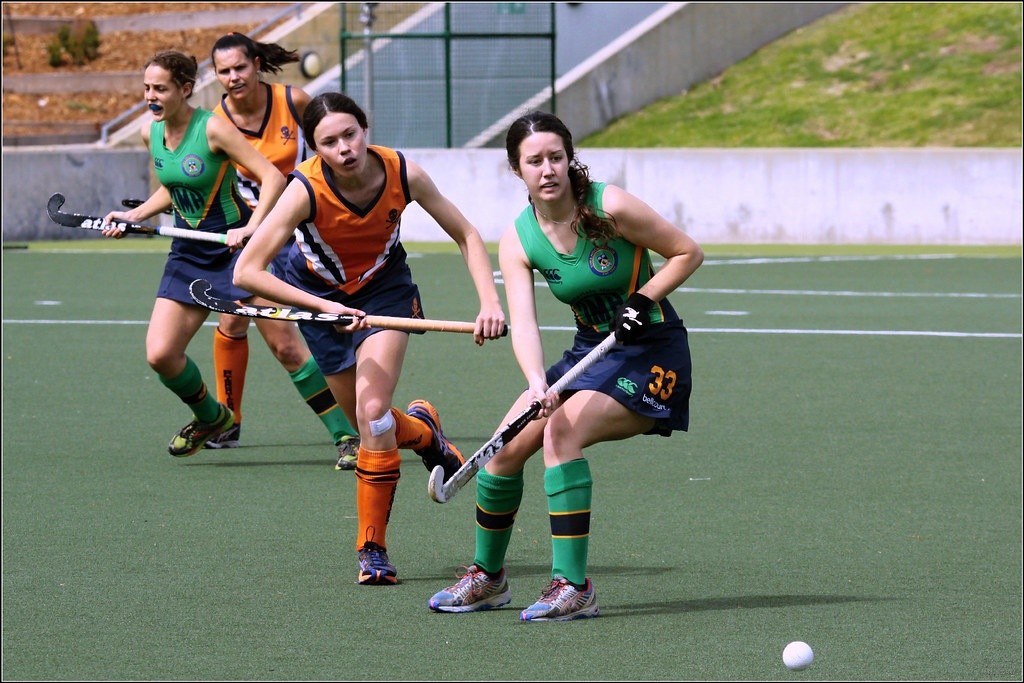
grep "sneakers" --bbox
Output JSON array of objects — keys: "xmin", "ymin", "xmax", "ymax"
[
  {"xmin": 168, "ymin": 402, "xmax": 235, "ymax": 459},
  {"xmin": 407, "ymin": 399, "xmax": 466, "ymax": 484},
  {"xmin": 205, "ymin": 423, "xmax": 241, "ymax": 448},
  {"xmin": 428, "ymin": 566, "xmax": 511, "ymax": 614},
  {"xmin": 335, "ymin": 435, "xmax": 361, "ymax": 470},
  {"xmin": 520, "ymin": 576, "xmax": 600, "ymax": 623},
  {"xmin": 357, "ymin": 541, "xmax": 398, "ymax": 585}
]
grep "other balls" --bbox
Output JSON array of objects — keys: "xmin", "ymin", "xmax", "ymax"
[{"xmin": 783, "ymin": 639, "xmax": 813, "ymax": 674}]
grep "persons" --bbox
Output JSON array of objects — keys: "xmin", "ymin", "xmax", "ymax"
[
  {"xmin": 203, "ymin": 31, "xmax": 362, "ymax": 449},
  {"xmin": 231, "ymin": 92, "xmax": 505, "ymax": 587},
  {"xmin": 423, "ymin": 112, "xmax": 709, "ymax": 622},
  {"xmin": 98, "ymin": 51, "xmax": 364, "ymax": 475}
]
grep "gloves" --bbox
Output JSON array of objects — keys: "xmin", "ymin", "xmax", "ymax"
[{"xmin": 610, "ymin": 293, "xmax": 656, "ymax": 345}]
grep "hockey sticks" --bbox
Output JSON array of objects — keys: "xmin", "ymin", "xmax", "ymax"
[
  {"xmin": 47, "ymin": 194, "xmax": 249, "ymax": 247},
  {"xmin": 122, "ymin": 197, "xmax": 174, "ymax": 215},
  {"xmin": 189, "ymin": 278, "xmax": 507, "ymax": 337},
  {"xmin": 429, "ymin": 332, "xmax": 619, "ymax": 504}
]
